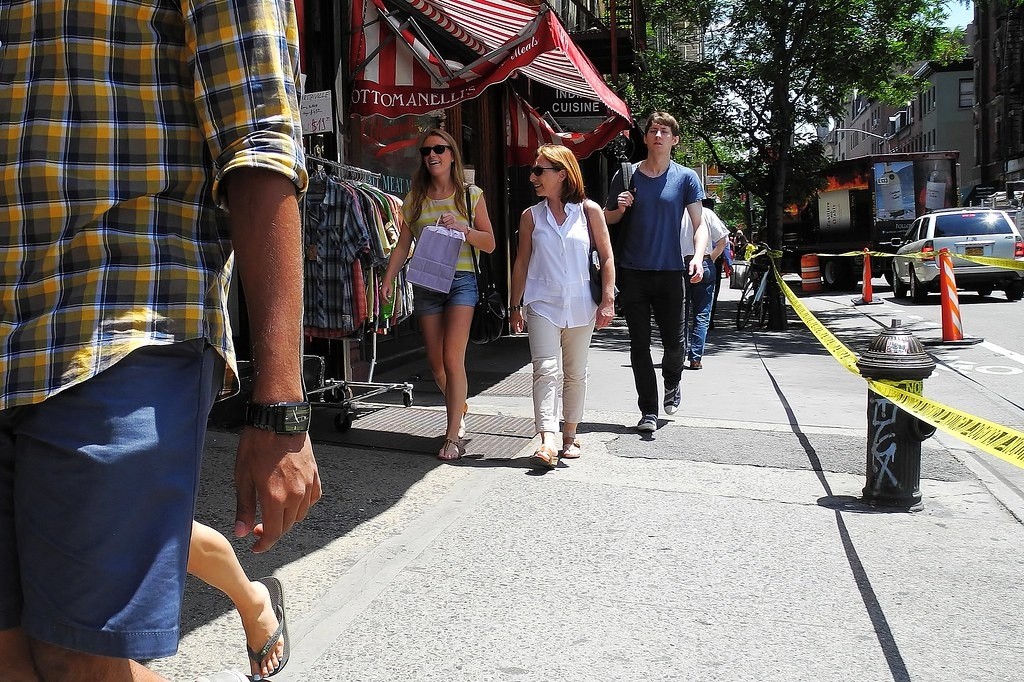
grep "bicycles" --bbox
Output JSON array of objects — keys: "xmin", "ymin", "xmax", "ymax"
[{"xmin": 737, "ymin": 241, "xmax": 793, "ymax": 330}]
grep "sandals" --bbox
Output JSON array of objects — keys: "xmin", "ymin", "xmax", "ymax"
[
  {"xmin": 438, "ymin": 439, "xmax": 462, "ymax": 461},
  {"xmin": 458, "ymin": 403, "xmax": 468, "ymax": 438}
]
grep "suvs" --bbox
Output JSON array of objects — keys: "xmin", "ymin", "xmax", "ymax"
[{"xmin": 891, "ymin": 207, "xmax": 1024, "ymax": 302}]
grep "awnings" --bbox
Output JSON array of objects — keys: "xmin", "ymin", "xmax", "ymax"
[{"xmin": 346, "ymin": 0, "xmax": 632, "ymax": 167}]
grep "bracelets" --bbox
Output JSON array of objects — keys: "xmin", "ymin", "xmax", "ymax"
[{"xmin": 509, "ymin": 305, "xmax": 520, "ymax": 312}]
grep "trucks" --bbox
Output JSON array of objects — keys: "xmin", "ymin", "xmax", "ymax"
[{"xmin": 756, "ymin": 150, "xmax": 962, "ymax": 292}]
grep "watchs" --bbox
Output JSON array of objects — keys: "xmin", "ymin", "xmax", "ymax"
[
  {"xmin": 242, "ymin": 399, "xmax": 312, "ymax": 436},
  {"xmin": 465, "ymin": 224, "xmax": 471, "ymax": 236}
]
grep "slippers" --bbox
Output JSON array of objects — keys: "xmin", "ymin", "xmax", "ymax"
[
  {"xmin": 246, "ymin": 576, "xmax": 290, "ymax": 679},
  {"xmin": 529, "ymin": 445, "xmax": 560, "ymax": 469},
  {"xmin": 562, "ymin": 438, "xmax": 580, "ymax": 458}
]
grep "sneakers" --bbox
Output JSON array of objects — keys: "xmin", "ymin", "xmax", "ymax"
[
  {"xmin": 664, "ymin": 382, "xmax": 681, "ymax": 416},
  {"xmin": 637, "ymin": 413, "xmax": 658, "ymax": 431}
]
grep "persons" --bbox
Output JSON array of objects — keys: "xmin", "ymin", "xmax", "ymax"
[
  {"xmin": 511, "ymin": 145, "xmax": 616, "ymax": 469},
  {"xmin": 602, "ymin": 111, "xmax": 708, "ymax": 432},
  {"xmin": 0, "ymin": 0, "xmax": 323, "ymax": 682},
  {"xmin": 679, "ymin": 197, "xmax": 749, "ymax": 370},
  {"xmin": 378, "ymin": 130, "xmax": 495, "ymax": 460}
]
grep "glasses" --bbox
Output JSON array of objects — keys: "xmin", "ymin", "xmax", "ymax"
[
  {"xmin": 419, "ymin": 145, "xmax": 453, "ymax": 156},
  {"xmin": 529, "ymin": 165, "xmax": 555, "ymax": 177}
]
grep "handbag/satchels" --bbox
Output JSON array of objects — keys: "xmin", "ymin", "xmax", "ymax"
[
  {"xmin": 478, "ymin": 291, "xmax": 504, "ymax": 344},
  {"xmin": 582, "ymin": 198, "xmax": 621, "ymax": 306},
  {"xmin": 404, "ymin": 214, "xmax": 466, "ymax": 294}
]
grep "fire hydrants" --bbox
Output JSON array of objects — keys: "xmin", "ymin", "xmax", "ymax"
[{"xmin": 855, "ymin": 319, "xmax": 937, "ymax": 511}]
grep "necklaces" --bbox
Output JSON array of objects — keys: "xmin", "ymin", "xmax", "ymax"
[{"xmin": 433, "ymin": 183, "xmax": 452, "ymax": 203}]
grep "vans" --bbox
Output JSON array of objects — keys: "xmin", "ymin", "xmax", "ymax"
[{"xmin": 1009, "ymin": 191, "xmax": 1024, "ymax": 237}]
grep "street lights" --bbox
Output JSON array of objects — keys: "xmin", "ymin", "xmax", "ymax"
[{"xmin": 829, "ymin": 128, "xmax": 890, "ymax": 154}]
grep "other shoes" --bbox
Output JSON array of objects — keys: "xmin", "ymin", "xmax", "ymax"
[{"xmin": 689, "ymin": 360, "xmax": 702, "ymax": 369}]
